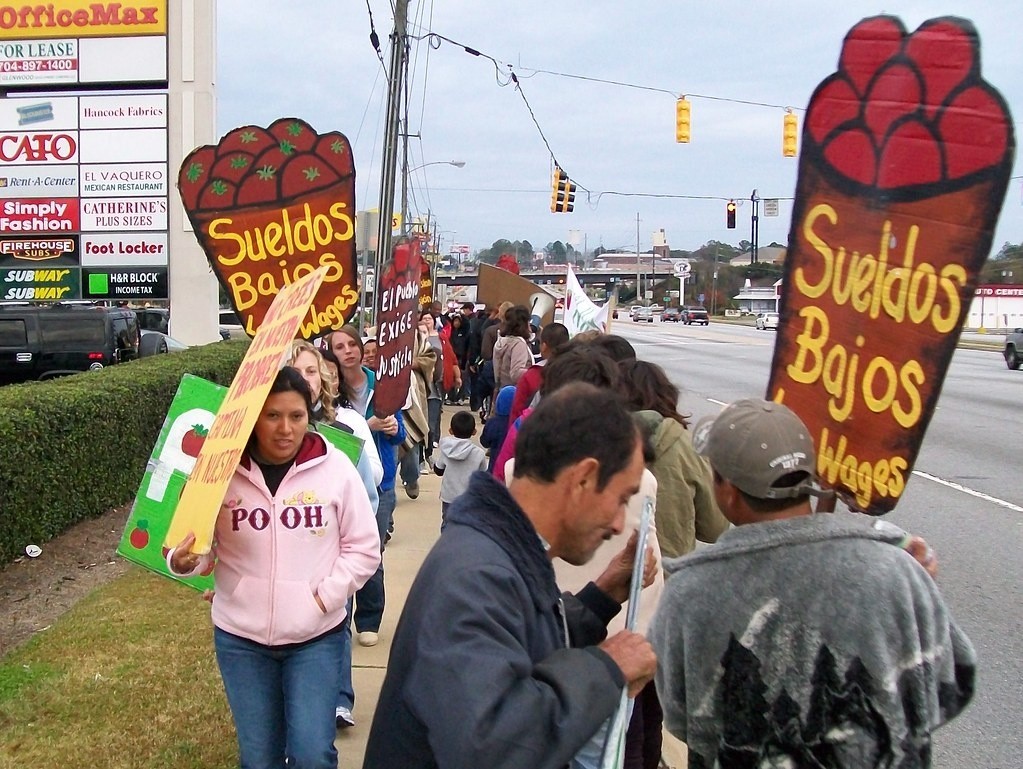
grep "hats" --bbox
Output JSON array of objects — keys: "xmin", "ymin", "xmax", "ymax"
[
  {"xmin": 691, "ymin": 398, "xmax": 834, "ymax": 501},
  {"xmin": 460, "ymin": 302, "xmax": 474, "ymax": 309}
]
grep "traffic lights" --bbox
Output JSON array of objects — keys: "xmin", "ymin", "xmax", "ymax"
[
  {"xmin": 550, "ymin": 169, "xmax": 567, "ymax": 213},
  {"xmin": 562, "ymin": 182, "xmax": 577, "ymax": 213},
  {"xmin": 726, "ymin": 202, "xmax": 736, "ymax": 229}
]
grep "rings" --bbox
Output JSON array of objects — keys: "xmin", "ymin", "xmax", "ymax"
[{"xmin": 187, "ymin": 556, "xmax": 194, "ymax": 563}]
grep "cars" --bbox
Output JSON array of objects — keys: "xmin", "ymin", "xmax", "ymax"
[
  {"xmin": 756, "ymin": 313, "xmax": 779, "ymax": 330},
  {"xmin": 1003, "ymin": 327, "xmax": 1023, "ymax": 370},
  {"xmin": 629, "ymin": 306, "xmax": 643, "ymax": 318},
  {"xmin": 660, "ymin": 308, "xmax": 679, "ymax": 323},
  {"xmin": 219, "ymin": 309, "xmax": 250, "ymax": 339},
  {"xmin": 134, "ymin": 308, "xmax": 231, "ymax": 339},
  {"xmin": 633, "ymin": 307, "xmax": 653, "ymax": 323}
]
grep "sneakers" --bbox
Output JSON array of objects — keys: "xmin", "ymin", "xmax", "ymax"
[
  {"xmin": 405, "ymin": 479, "xmax": 419, "ymax": 499},
  {"xmin": 358, "ymin": 631, "xmax": 378, "ymax": 647},
  {"xmin": 335, "ymin": 706, "xmax": 355, "ymax": 728}
]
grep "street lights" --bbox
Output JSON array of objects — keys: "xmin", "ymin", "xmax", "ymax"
[{"xmin": 400, "ymin": 160, "xmax": 466, "ymax": 236}]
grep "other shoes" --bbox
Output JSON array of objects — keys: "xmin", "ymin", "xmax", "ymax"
[
  {"xmin": 420, "ymin": 456, "xmax": 435, "ymax": 474},
  {"xmin": 433, "ymin": 441, "xmax": 438, "ymax": 448}
]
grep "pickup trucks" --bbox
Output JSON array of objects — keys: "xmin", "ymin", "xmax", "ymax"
[
  {"xmin": 683, "ymin": 306, "xmax": 710, "ymax": 326},
  {"xmin": 650, "ymin": 304, "xmax": 665, "ymax": 312}
]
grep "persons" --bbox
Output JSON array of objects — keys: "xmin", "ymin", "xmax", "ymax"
[
  {"xmin": 121, "ymin": 303, "xmax": 129, "ymax": 308},
  {"xmin": 285, "ymin": 301, "xmax": 731, "ymax": 769},
  {"xmin": 645, "ymin": 399, "xmax": 979, "ymax": 769},
  {"xmin": 166, "ymin": 365, "xmax": 381, "ymax": 769}
]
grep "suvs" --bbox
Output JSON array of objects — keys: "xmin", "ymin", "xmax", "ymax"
[{"xmin": 0, "ymin": 302, "xmax": 169, "ymax": 389}]
